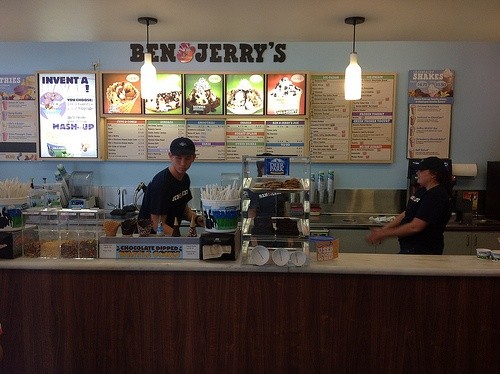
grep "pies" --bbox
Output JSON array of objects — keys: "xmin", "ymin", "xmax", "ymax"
[{"xmin": 264, "ymin": 178, "xmax": 300, "ymax": 189}]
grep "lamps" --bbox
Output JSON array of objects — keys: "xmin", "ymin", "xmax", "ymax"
[
  {"xmin": 343, "ymin": 17, "xmax": 365, "ymax": 101},
  {"xmin": 138, "ymin": 17, "xmax": 159, "ymax": 100}
]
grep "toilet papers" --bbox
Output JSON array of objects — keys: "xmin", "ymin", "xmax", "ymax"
[{"xmin": 452, "ymin": 164, "xmax": 478, "ymax": 176}]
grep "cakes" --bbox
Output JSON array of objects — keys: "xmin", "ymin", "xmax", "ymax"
[{"xmin": 145, "ymin": 86, "xmax": 183, "ymax": 111}]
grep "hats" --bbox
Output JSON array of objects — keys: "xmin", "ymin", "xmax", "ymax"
[
  {"xmin": 170, "ymin": 137, "xmax": 195, "ymax": 155},
  {"xmin": 411, "ymin": 157, "xmax": 444, "ymax": 172}
]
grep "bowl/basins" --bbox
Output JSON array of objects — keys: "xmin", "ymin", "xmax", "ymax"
[
  {"xmin": 475, "ymin": 248, "xmax": 491, "ymax": 260},
  {"xmin": 490, "ymin": 250, "xmax": 500, "ymax": 262}
]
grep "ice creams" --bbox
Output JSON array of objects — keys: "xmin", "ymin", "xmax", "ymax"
[
  {"xmin": 186, "ymin": 77, "xmax": 302, "ymax": 115},
  {"xmin": 106, "ymin": 81, "xmax": 138, "ymax": 112}
]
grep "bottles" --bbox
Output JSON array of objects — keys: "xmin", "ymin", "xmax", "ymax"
[{"xmin": 309, "ymin": 170, "xmax": 334, "ymax": 204}]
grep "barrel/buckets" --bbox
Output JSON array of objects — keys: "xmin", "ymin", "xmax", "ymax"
[
  {"xmin": 201, "ymin": 198, "xmax": 241, "ymax": 233},
  {"xmin": 0, "ymin": 196, "xmax": 29, "ymax": 231}
]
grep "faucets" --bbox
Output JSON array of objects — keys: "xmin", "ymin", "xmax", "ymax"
[{"xmin": 106, "ymin": 189, "xmax": 123, "ymax": 209}]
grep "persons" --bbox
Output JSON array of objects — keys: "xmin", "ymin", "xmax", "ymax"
[
  {"xmin": 366, "ymin": 157, "xmax": 453, "ymax": 255},
  {"xmin": 138, "ymin": 137, "xmax": 204, "ymax": 236}
]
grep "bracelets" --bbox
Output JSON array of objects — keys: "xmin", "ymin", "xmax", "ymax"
[{"xmin": 195, "ymin": 216, "xmax": 200, "ymax": 225}]
